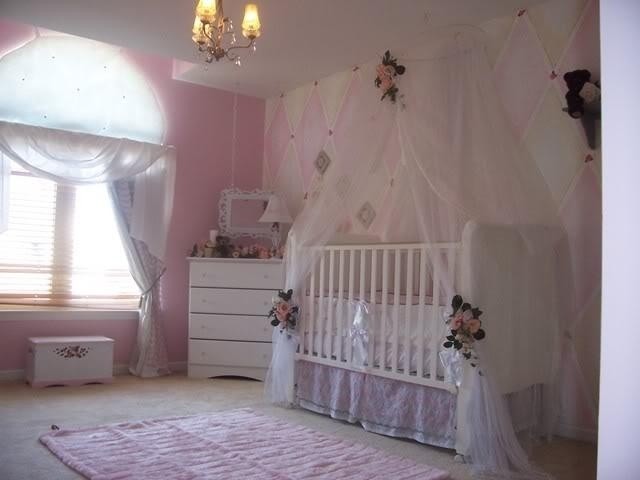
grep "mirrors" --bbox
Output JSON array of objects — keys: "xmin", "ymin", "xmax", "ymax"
[{"xmin": 216, "ymin": 183, "xmax": 283, "ymax": 241}]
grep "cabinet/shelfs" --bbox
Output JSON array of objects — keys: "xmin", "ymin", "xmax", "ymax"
[{"xmin": 185, "ymin": 255, "xmax": 287, "ymax": 379}]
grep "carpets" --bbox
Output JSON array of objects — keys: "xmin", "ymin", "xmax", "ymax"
[{"xmin": 39, "ymin": 406, "xmax": 450, "ymax": 480}]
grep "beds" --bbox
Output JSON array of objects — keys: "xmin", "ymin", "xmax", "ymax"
[{"xmin": 277, "ymin": 219, "xmax": 553, "ymax": 465}]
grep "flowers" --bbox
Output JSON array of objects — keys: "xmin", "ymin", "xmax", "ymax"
[
  {"xmin": 441, "ymin": 293, "xmax": 484, "ymax": 364},
  {"xmin": 268, "ymin": 286, "xmax": 300, "ymax": 333},
  {"xmin": 374, "ymin": 47, "xmax": 406, "ymax": 103}
]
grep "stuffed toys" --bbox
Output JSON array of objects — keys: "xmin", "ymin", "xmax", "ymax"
[
  {"xmin": 214, "ymin": 235, "xmax": 240, "ymax": 257},
  {"xmin": 563, "ymin": 69, "xmax": 591, "ymax": 119}
]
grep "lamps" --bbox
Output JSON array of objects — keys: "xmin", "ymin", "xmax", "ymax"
[{"xmin": 184, "ymin": 0, "xmax": 265, "ymax": 67}]
